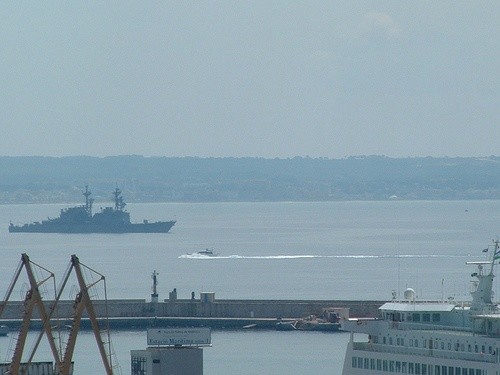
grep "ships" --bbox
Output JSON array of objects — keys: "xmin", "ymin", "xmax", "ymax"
[
  {"xmin": 337, "ymin": 239, "xmax": 500, "ymax": 375},
  {"xmin": 9, "ymin": 184, "xmax": 175, "ymax": 234}
]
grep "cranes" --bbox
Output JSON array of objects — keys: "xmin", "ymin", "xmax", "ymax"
[
  {"xmin": 23, "ymin": 256, "xmax": 121, "ymax": 375},
  {"xmin": 1, "ymin": 253, "xmax": 61, "ymax": 375}
]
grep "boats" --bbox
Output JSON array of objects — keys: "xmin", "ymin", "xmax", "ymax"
[{"xmin": 197, "ymin": 248, "xmax": 214, "ymax": 257}]
